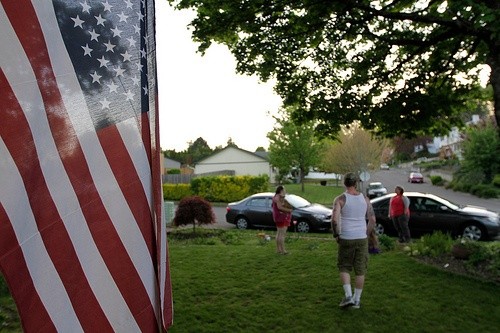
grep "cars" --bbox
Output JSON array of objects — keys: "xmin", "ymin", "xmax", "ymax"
[
  {"xmin": 366, "ymin": 182, "xmax": 388, "ymax": 199},
  {"xmin": 407, "ymin": 172, "xmax": 424, "ymax": 184},
  {"xmin": 381, "ymin": 163, "xmax": 389, "ymax": 170},
  {"xmin": 224, "ymin": 192, "xmax": 333, "ymax": 233},
  {"xmin": 365, "ymin": 191, "xmax": 500, "ymax": 241}
]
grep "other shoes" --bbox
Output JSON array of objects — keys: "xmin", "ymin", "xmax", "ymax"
[
  {"xmin": 352, "ymin": 301, "xmax": 361, "ymax": 308},
  {"xmin": 339, "ymin": 295, "xmax": 355, "ymax": 308}
]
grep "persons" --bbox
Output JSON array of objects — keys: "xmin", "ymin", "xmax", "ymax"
[
  {"xmin": 271, "ymin": 186, "xmax": 295, "ymax": 254},
  {"xmin": 331, "ymin": 173, "xmax": 376, "ymax": 308},
  {"xmin": 388, "ymin": 186, "xmax": 410, "ymax": 243}
]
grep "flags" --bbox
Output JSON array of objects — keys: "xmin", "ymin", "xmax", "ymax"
[{"xmin": 1, "ymin": 0, "xmax": 173, "ymax": 333}]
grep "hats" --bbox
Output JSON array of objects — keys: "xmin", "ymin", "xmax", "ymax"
[{"xmin": 344, "ymin": 173, "xmax": 357, "ymax": 181}]
